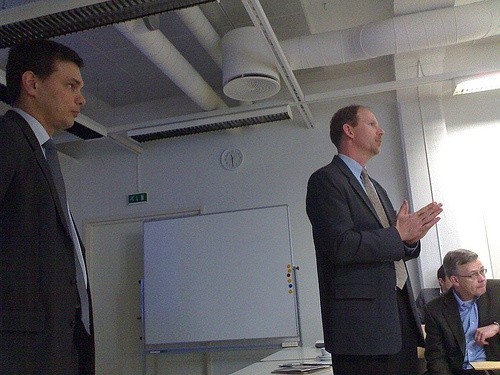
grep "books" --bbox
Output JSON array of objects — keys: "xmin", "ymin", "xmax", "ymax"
[{"xmin": 271, "ymin": 361, "xmax": 332, "ymax": 374}]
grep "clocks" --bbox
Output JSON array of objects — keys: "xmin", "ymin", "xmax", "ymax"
[{"xmin": 220, "ymin": 146, "xmax": 244, "ymax": 171}]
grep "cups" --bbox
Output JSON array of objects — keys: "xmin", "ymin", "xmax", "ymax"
[{"xmin": 321, "ymin": 347, "xmax": 330, "ymax": 356}]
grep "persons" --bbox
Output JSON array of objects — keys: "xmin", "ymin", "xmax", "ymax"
[
  {"xmin": 0, "ymin": 38, "xmax": 95, "ymax": 375},
  {"xmin": 306, "ymin": 105, "xmax": 442, "ymax": 375},
  {"xmin": 425, "ymin": 249, "xmax": 500, "ymax": 375},
  {"xmin": 415, "ymin": 265, "xmax": 453, "ymax": 325}
]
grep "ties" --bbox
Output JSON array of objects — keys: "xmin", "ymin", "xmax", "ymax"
[
  {"xmin": 359, "ymin": 169, "xmax": 409, "ymax": 291},
  {"xmin": 42, "ymin": 136, "xmax": 91, "ymax": 335}
]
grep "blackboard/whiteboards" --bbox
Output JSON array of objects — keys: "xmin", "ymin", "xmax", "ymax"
[{"xmin": 141, "ymin": 204, "xmax": 303, "ymax": 354}]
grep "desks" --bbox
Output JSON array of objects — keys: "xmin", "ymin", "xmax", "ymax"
[{"xmin": 229, "ymin": 347, "xmax": 333, "ymax": 375}]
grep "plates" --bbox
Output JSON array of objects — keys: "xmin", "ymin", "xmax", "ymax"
[{"xmin": 317, "ymin": 353, "xmax": 332, "ymax": 361}]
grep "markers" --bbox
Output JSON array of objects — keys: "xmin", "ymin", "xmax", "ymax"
[{"xmin": 149, "ymin": 351, "xmax": 163, "ymax": 354}]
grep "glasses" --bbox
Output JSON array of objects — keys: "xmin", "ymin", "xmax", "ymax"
[{"xmin": 454, "ymin": 269, "xmax": 487, "ymax": 279}]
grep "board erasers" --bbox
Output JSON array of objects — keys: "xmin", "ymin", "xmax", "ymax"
[{"xmin": 282, "ymin": 342, "xmax": 299, "ymax": 348}]
